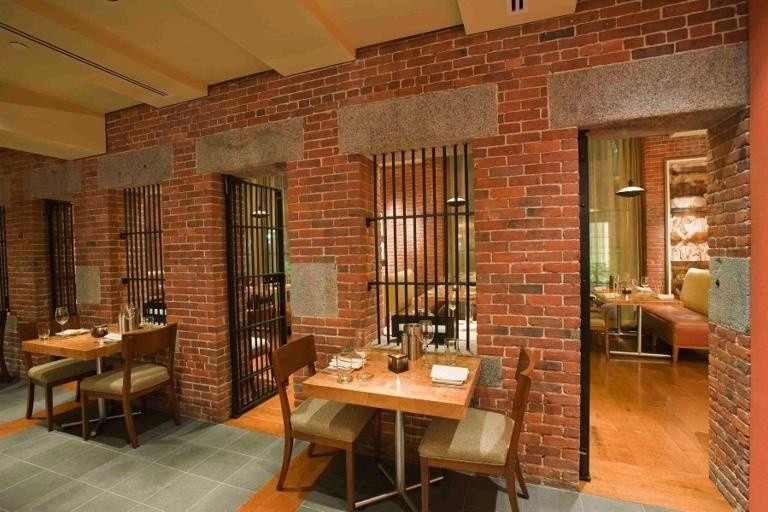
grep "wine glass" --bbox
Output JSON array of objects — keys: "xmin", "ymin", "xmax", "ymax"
[
  {"xmin": 54, "ymin": 307, "xmax": 70, "ymax": 332},
  {"xmin": 354, "ymin": 329, "xmax": 371, "ymax": 383},
  {"xmin": 415, "ymin": 320, "xmax": 435, "ymax": 359}
]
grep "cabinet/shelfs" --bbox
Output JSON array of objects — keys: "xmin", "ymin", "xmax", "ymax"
[{"xmin": 664, "ymin": 154, "xmax": 713, "ymax": 292}]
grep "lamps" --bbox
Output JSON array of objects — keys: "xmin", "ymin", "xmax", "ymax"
[
  {"xmin": 613, "ymin": 133, "xmax": 648, "ymax": 200},
  {"xmin": 447, "ymin": 144, "xmax": 466, "ymax": 206},
  {"xmin": 249, "ymin": 185, "xmax": 272, "ymax": 218}
]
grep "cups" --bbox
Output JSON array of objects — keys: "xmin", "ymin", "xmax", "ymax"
[
  {"xmin": 37, "ymin": 321, "xmax": 49, "ymax": 341},
  {"xmin": 444, "ymin": 337, "xmax": 459, "ymax": 366},
  {"xmin": 336, "ymin": 351, "xmax": 353, "ymax": 384}
]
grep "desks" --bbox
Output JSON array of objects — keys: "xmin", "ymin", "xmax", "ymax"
[
  {"xmin": 594, "ymin": 284, "xmax": 683, "ymax": 359},
  {"xmin": 421, "ymin": 279, "xmax": 478, "ymax": 321}
]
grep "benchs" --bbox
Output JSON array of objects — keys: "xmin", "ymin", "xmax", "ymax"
[
  {"xmin": 633, "ymin": 264, "xmax": 712, "ymax": 362},
  {"xmin": 387, "ymin": 266, "xmax": 445, "ymax": 319}
]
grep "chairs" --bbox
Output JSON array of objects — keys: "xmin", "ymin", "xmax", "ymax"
[
  {"xmin": 418, "ymin": 345, "xmax": 536, "ymax": 512},
  {"xmin": 16, "ymin": 316, "xmax": 183, "ymax": 451},
  {"xmin": 270, "ymin": 333, "xmax": 383, "ymax": 511}
]
